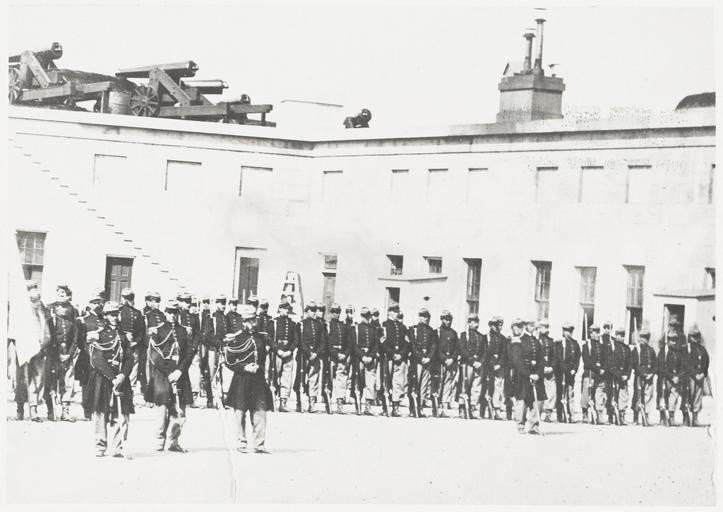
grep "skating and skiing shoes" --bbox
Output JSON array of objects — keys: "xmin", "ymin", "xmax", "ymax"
[{"xmin": 515, "ymin": 422, "xmax": 549, "ymax": 435}]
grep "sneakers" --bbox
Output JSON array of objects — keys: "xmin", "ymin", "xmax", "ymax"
[{"xmin": 237, "ymin": 446, "xmax": 271, "ymax": 452}]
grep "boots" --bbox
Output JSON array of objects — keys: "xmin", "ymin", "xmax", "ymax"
[
  {"xmin": 6, "ymin": 413, "xmax": 23, "ymax": 420},
  {"xmin": 29, "ymin": 406, "xmax": 42, "ymax": 422},
  {"xmin": 62, "ymin": 406, "xmax": 76, "ymax": 423},
  {"xmin": 279, "ymin": 399, "xmax": 505, "ymax": 421},
  {"xmin": 46, "ymin": 398, "xmax": 58, "ymax": 420},
  {"xmin": 553, "ymin": 411, "xmax": 705, "ymax": 427}
]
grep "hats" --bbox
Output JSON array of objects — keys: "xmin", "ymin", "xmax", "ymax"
[
  {"xmin": 145, "ymin": 291, "xmax": 161, "ymax": 303},
  {"xmin": 247, "ymin": 294, "xmax": 403, "ymax": 319},
  {"xmin": 103, "ymin": 300, "xmax": 121, "ymax": 314},
  {"xmin": 415, "ymin": 309, "xmax": 704, "ymax": 341},
  {"xmin": 89, "ymin": 293, "xmax": 103, "ymax": 303},
  {"xmin": 165, "ymin": 301, "xmax": 180, "ymax": 313},
  {"xmin": 122, "ymin": 288, "xmax": 135, "ymax": 299},
  {"xmin": 176, "ymin": 293, "xmax": 240, "ymax": 308}
]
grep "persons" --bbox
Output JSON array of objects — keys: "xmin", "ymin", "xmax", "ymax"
[
  {"xmin": 7, "ymin": 281, "xmax": 710, "ymax": 457},
  {"xmin": 345, "ymin": 109, "xmax": 372, "ymax": 128}
]
